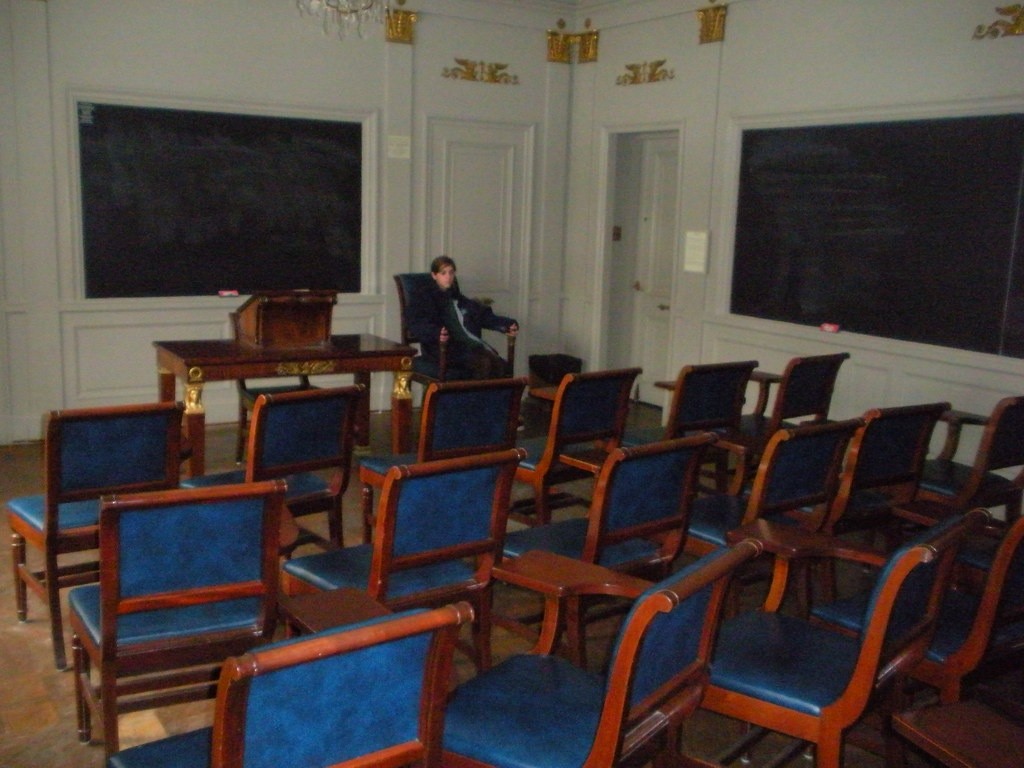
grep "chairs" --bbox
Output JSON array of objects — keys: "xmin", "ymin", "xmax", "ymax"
[
  {"xmin": 229, "ymin": 311, "xmax": 323, "ymax": 462},
  {"xmin": 808, "ymin": 514, "xmax": 1024, "ymax": 706},
  {"xmin": 682, "ymin": 413, "xmax": 865, "ymax": 621},
  {"xmin": 916, "ymin": 396, "xmax": 1024, "ymax": 507},
  {"xmin": 441, "ymin": 537, "xmax": 764, "ymax": 768},
  {"xmin": 68, "ymin": 479, "xmax": 289, "ymax": 768},
  {"xmin": 177, "ymin": 383, "xmax": 365, "ymax": 560},
  {"xmin": 506, "ymin": 366, "xmax": 643, "ymax": 528},
  {"xmin": 109, "ymin": 600, "xmax": 474, "ymax": 768},
  {"xmin": 6, "ymin": 400, "xmax": 187, "ymax": 670},
  {"xmin": 358, "ymin": 375, "xmax": 531, "ymax": 544},
  {"xmin": 782, "ymin": 401, "xmax": 952, "ymax": 535},
  {"xmin": 393, "ymin": 273, "xmax": 517, "ymax": 404},
  {"xmin": 741, "ymin": 352, "xmax": 850, "ymax": 455},
  {"xmin": 650, "ymin": 506, "xmax": 993, "ymax": 768},
  {"xmin": 280, "ymin": 446, "xmax": 529, "ymax": 673},
  {"xmin": 491, "ymin": 431, "xmax": 721, "ymax": 667},
  {"xmin": 621, "ymin": 360, "xmax": 759, "ymax": 495}
]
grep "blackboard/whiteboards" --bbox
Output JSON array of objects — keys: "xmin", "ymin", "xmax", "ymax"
[
  {"xmin": 67, "ymin": 91, "xmax": 381, "ymax": 304},
  {"xmin": 705, "ymin": 95, "xmax": 1024, "ymax": 377}
]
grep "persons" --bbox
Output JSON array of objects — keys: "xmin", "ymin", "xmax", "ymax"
[{"xmin": 405, "ymin": 253, "xmax": 529, "ymax": 433}]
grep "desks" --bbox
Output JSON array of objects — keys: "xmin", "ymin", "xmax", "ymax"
[{"xmin": 151, "ymin": 290, "xmax": 419, "ymax": 477}]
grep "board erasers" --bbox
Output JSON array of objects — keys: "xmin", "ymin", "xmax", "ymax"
[
  {"xmin": 819, "ymin": 322, "xmax": 840, "ymax": 333},
  {"xmin": 218, "ymin": 290, "xmax": 239, "ymax": 297}
]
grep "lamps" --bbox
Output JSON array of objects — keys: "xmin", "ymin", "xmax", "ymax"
[{"xmin": 296, "ymin": 0, "xmax": 385, "ymax": 44}]
